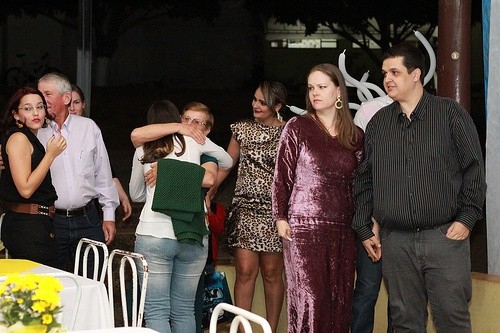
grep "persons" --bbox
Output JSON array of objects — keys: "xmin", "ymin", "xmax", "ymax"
[
  {"xmin": 271, "ymin": 63, "xmax": 381, "ymax": 333},
  {"xmin": 130, "ymin": 101, "xmax": 233, "ymax": 333},
  {"xmin": 0, "ymin": 86, "xmax": 67, "ymax": 267},
  {"xmin": 351, "ymin": 92, "xmax": 393, "ymax": 333},
  {"xmin": 205, "ymin": 82, "xmax": 288, "ymax": 333},
  {"xmin": 350, "ymin": 44, "xmax": 487, "ymax": 333},
  {"xmin": 67, "ymin": 86, "xmax": 132, "ymax": 221},
  {"xmin": 0, "ymin": 73, "xmax": 119, "ymax": 281}
]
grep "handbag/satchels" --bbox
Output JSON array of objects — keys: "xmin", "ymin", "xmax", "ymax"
[{"xmin": 202, "ymin": 272, "xmax": 235, "ymax": 330}]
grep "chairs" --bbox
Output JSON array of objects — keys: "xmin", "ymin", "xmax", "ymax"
[
  {"xmin": 208, "ymin": 303, "xmax": 272, "ymax": 333},
  {"xmin": 73, "ymin": 237, "xmax": 109, "ymax": 283},
  {"xmin": 107, "ymin": 249, "xmax": 149, "ymax": 328}
]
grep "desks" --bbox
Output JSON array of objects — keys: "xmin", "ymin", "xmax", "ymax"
[{"xmin": 0, "ymin": 258, "xmax": 113, "ymax": 333}]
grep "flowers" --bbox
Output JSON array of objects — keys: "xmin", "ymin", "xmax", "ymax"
[{"xmin": 0, "ymin": 272, "xmax": 65, "ymax": 333}]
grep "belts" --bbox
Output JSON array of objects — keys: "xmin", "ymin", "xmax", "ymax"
[
  {"xmin": 7, "ymin": 202, "xmax": 56, "ymax": 218},
  {"xmin": 56, "ymin": 200, "xmax": 95, "ymax": 217}
]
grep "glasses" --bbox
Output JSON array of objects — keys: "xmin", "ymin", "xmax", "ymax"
[
  {"xmin": 180, "ymin": 115, "xmax": 207, "ymax": 126},
  {"xmin": 18, "ymin": 104, "xmax": 47, "ymax": 112}
]
